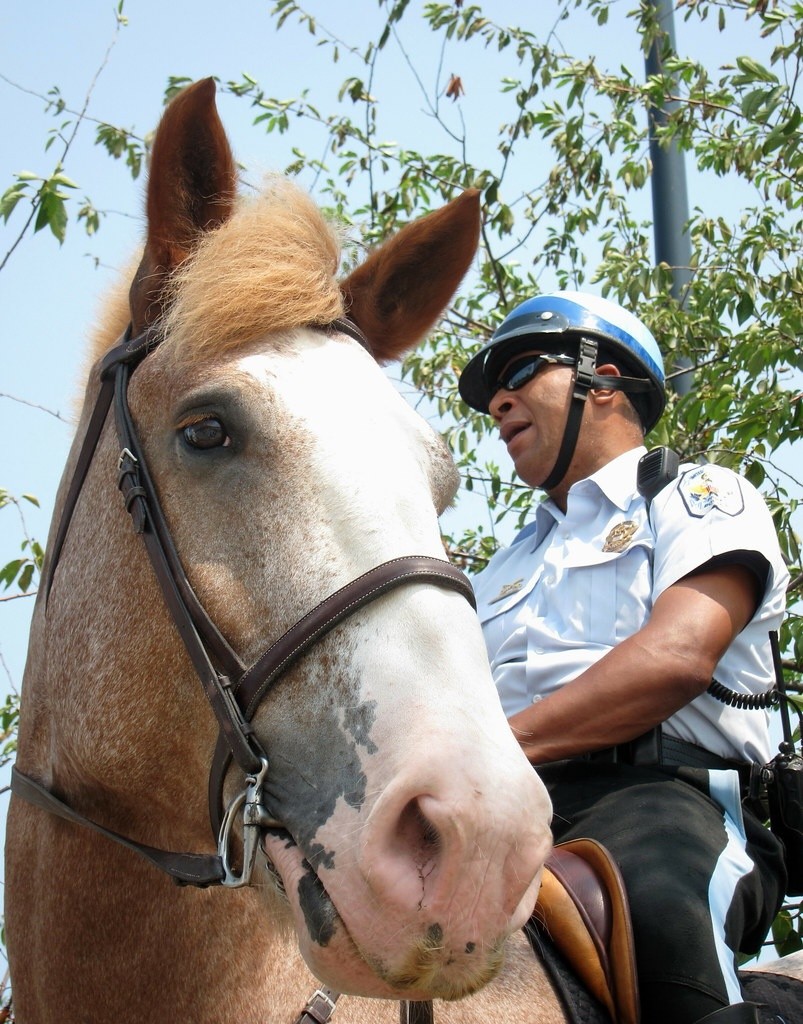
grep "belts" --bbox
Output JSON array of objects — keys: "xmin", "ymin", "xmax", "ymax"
[{"xmin": 596, "ymin": 731, "xmax": 727, "ymax": 770}]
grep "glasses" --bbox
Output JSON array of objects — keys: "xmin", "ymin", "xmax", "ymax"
[{"xmin": 489, "ymin": 353, "xmax": 575, "ymax": 400}]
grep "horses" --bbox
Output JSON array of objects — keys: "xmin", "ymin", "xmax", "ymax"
[{"xmin": 0, "ymin": 74, "xmax": 571, "ymax": 1023}]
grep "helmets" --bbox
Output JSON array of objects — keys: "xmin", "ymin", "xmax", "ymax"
[{"xmin": 458, "ymin": 290, "xmax": 669, "ymax": 436}]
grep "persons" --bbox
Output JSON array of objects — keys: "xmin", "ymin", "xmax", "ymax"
[{"xmin": 457, "ymin": 289, "xmax": 792, "ymax": 1024}]
